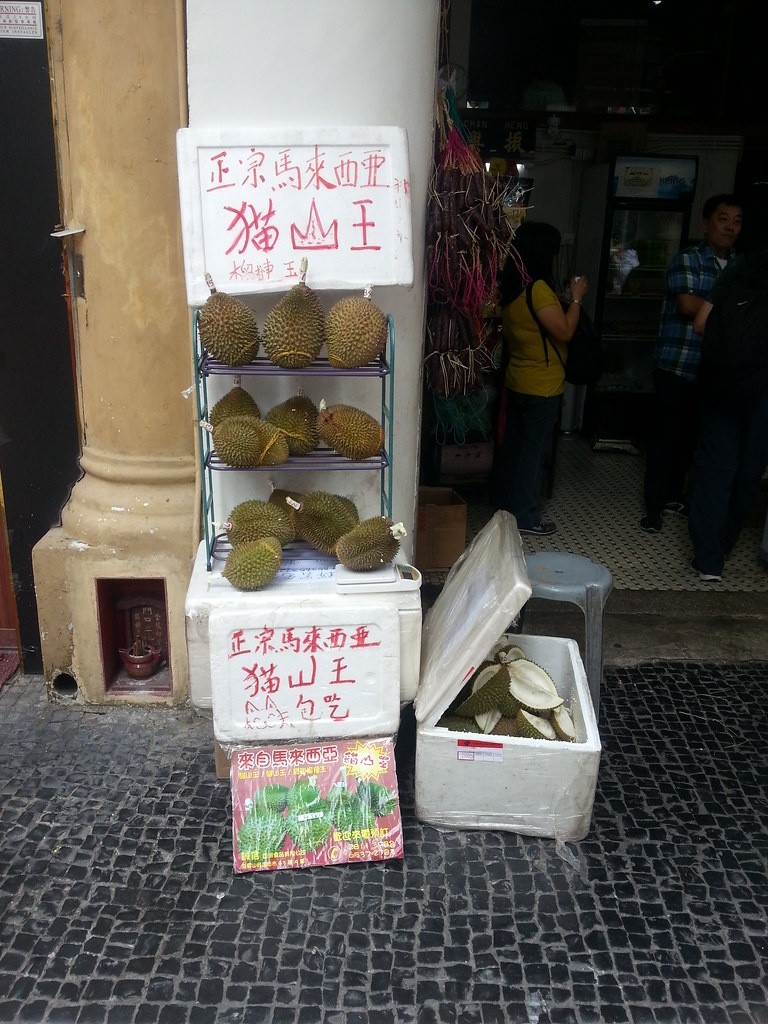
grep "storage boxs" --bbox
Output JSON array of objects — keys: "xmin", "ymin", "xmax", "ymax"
[
  {"xmin": 184, "ymin": 538, "xmax": 423, "ymax": 710},
  {"xmin": 403, "ymin": 509, "xmax": 594, "ymax": 840},
  {"xmin": 410, "ymin": 485, "xmax": 468, "ymax": 569},
  {"xmin": 436, "ymin": 432, "xmax": 494, "ymax": 473}
]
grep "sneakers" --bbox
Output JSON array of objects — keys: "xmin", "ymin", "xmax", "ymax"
[
  {"xmin": 691, "ymin": 558, "xmax": 722, "ymax": 582},
  {"xmin": 640, "ymin": 517, "xmax": 661, "ymax": 532},
  {"xmin": 517, "ymin": 521, "xmax": 556, "ymax": 535}
]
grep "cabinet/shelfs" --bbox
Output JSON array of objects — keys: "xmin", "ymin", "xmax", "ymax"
[
  {"xmin": 190, "ymin": 305, "xmax": 395, "ymax": 561},
  {"xmin": 593, "ymin": 201, "xmax": 693, "ymax": 385}
]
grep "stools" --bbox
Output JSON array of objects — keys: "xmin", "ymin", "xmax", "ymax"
[{"xmin": 505, "ymin": 548, "xmax": 616, "ymax": 724}]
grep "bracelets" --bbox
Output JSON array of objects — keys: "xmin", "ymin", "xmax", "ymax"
[{"xmin": 570, "ymin": 299, "xmax": 582, "ymax": 305}]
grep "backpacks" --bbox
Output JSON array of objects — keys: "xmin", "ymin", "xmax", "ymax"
[{"xmin": 525, "ymin": 278, "xmax": 607, "ymax": 386}]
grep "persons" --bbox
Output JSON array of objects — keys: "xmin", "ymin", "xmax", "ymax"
[
  {"xmin": 497, "ymin": 221, "xmax": 589, "ymax": 534},
  {"xmin": 638, "ymin": 194, "xmax": 768, "ymax": 582}
]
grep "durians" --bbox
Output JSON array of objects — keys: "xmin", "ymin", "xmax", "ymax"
[{"xmin": 196, "ymin": 285, "xmax": 401, "ymax": 591}]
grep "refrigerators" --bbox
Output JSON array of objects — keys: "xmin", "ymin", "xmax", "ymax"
[{"xmin": 565, "ymin": 150, "xmax": 702, "ymax": 451}]
